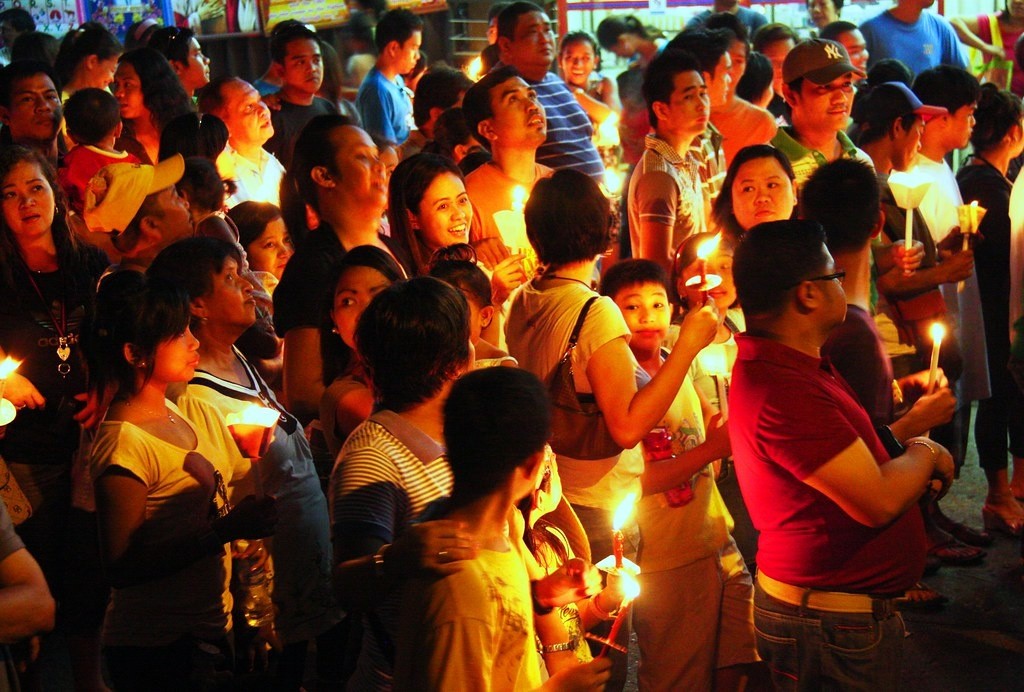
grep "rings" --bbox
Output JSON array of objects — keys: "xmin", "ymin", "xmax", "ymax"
[
  {"xmin": 19, "ymin": 403, "xmax": 26, "ymax": 409},
  {"xmin": 438, "ymin": 549, "xmax": 448, "ymax": 560},
  {"xmin": 258, "ymin": 548, "xmax": 264, "ymax": 552}
]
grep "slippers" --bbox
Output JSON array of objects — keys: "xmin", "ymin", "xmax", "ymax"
[
  {"xmin": 923, "ymin": 535, "xmax": 987, "ymax": 562},
  {"xmin": 922, "ymin": 556, "xmax": 942, "ymax": 577},
  {"xmin": 897, "ymin": 581, "xmax": 950, "ymax": 609},
  {"xmin": 983, "ymin": 485, "xmax": 1024, "ymax": 537},
  {"xmin": 944, "ymin": 522, "xmax": 996, "ymax": 544}
]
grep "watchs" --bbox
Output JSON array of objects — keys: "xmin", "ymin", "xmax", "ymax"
[{"xmin": 373, "ymin": 544, "xmax": 392, "ymax": 569}]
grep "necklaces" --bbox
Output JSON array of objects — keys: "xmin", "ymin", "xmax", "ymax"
[
  {"xmin": 540, "ymin": 273, "xmax": 590, "ymax": 289},
  {"xmin": 22, "ymin": 262, "xmax": 72, "ymax": 375},
  {"xmin": 127, "ymin": 400, "xmax": 175, "ymax": 424}
]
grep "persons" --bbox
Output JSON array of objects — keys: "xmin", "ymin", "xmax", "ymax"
[{"xmin": 0, "ymin": 0, "xmax": 1024, "ymax": 692}]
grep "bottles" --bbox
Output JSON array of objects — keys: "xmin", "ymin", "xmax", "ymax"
[{"xmin": 243, "ymin": 569, "xmax": 272, "ymax": 627}]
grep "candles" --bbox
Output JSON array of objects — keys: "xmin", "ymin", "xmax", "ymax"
[
  {"xmin": 696, "ymin": 230, "xmax": 723, "ymax": 306},
  {"xmin": 612, "ymin": 492, "xmax": 634, "ymax": 569},
  {"xmin": 258, "ymin": 420, "xmax": 278, "ymax": 457},
  {"xmin": 717, "ymin": 374, "xmax": 728, "ymax": 423},
  {"xmin": 906, "ymin": 208, "xmax": 913, "ymax": 274},
  {"xmin": 957, "ymin": 200, "xmax": 978, "ymax": 294},
  {"xmin": 599, "ymin": 569, "xmax": 640, "ymax": 657},
  {"xmin": 511, "ymin": 186, "xmax": 527, "ymax": 256},
  {"xmin": 0, "ymin": 356, "xmax": 22, "ymax": 404},
  {"xmin": 921, "ymin": 321, "xmax": 945, "ymax": 438}
]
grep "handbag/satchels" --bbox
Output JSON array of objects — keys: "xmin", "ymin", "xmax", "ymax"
[
  {"xmin": 969, "ymin": 14, "xmax": 1014, "ymax": 95},
  {"xmin": 69, "ymin": 424, "xmax": 101, "ymax": 513},
  {"xmin": 543, "ymin": 297, "xmax": 625, "ymax": 460},
  {"xmin": 878, "ymin": 423, "xmax": 906, "ymax": 459}
]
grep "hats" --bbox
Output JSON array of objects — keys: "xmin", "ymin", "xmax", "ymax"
[
  {"xmin": 868, "ymin": 58, "xmax": 914, "ymax": 93},
  {"xmin": 81, "ymin": 152, "xmax": 186, "ymax": 239},
  {"xmin": 781, "ymin": 39, "xmax": 867, "ymax": 87},
  {"xmin": 852, "ymin": 81, "xmax": 947, "ymax": 132}
]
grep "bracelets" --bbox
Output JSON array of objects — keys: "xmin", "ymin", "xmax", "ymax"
[
  {"xmin": 572, "ymin": 88, "xmax": 584, "ymax": 99},
  {"xmin": 538, "ymin": 640, "xmax": 575, "ymax": 653},
  {"xmin": 529, "ymin": 579, "xmax": 553, "ymax": 616},
  {"xmin": 908, "ymin": 441, "xmax": 937, "ymax": 464}
]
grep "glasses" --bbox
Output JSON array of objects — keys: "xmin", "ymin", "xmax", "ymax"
[
  {"xmin": 274, "ymin": 23, "xmax": 305, "ymax": 36},
  {"xmin": 805, "ymin": 268, "xmax": 846, "ymax": 284},
  {"xmin": 165, "ymin": 26, "xmax": 181, "ymax": 62}
]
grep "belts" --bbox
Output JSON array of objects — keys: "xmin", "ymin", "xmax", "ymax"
[{"xmin": 755, "ymin": 566, "xmax": 876, "ymax": 613}]
